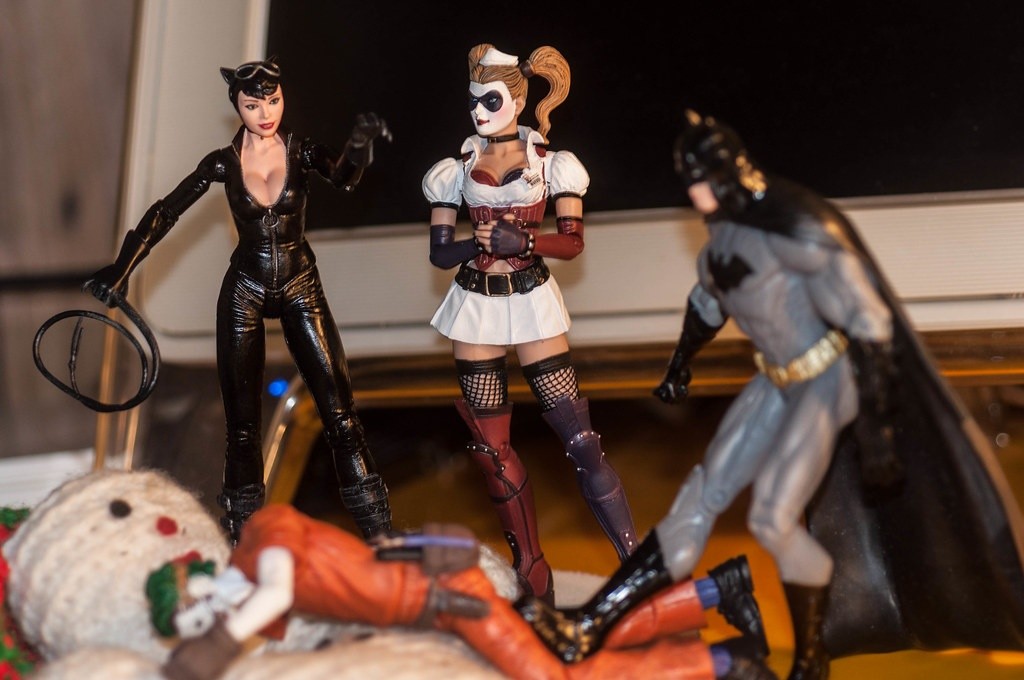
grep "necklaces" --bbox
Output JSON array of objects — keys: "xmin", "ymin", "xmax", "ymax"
[{"xmin": 488, "ymin": 130, "xmax": 520, "ymax": 144}]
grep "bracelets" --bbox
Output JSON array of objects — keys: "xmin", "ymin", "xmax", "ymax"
[{"xmin": 518, "ymin": 232, "xmax": 535, "ymax": 260}]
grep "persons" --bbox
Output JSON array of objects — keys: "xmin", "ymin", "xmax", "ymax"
[
  {"xmin": 422, "ymin": 41, "xmax": 639, "ymax": 604},
  {"xmin": 84, "ymin": 55, "xmax": 395, "ymax": 547},
  {"xmin": 511, "ymin": 115, "xmax": 891, "ymax": 680},
  {"xmin": 145, "ymin": 503, "xmax": 783, "ymax": 680}
]
